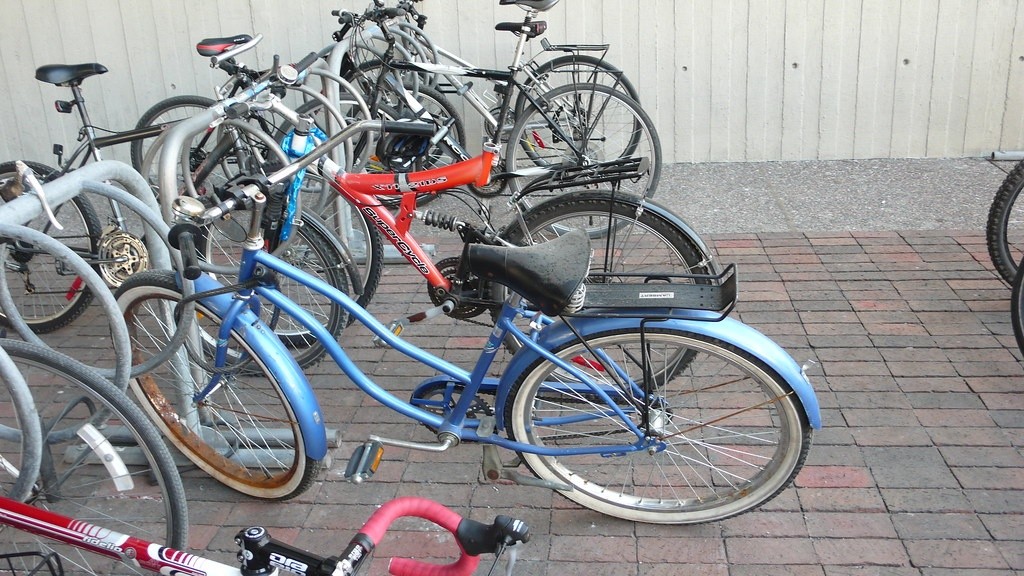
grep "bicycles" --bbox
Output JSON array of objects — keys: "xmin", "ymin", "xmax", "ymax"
[
  {"xmin": 984, "ymin": 151, "xmax": 1024, "ymax": 359},
  {"xmin": 0, "ymin": 0, "xmax": 824, "ymax": 576}
]
